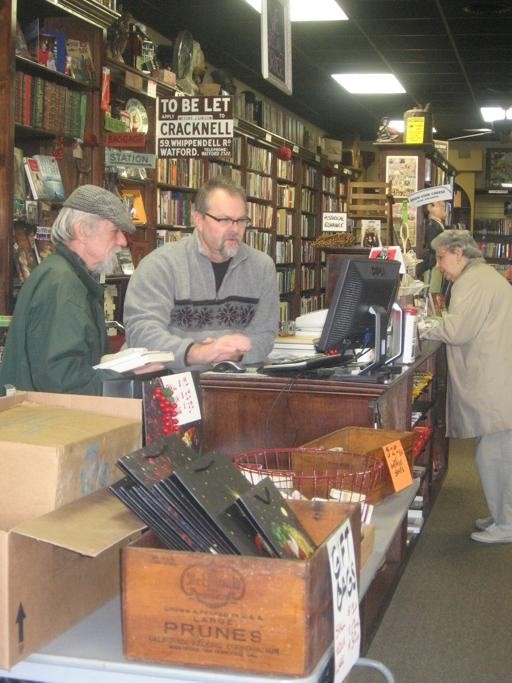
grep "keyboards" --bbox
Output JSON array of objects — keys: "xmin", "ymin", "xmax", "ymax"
[{"xmin": 256, "ymin": 353, "xmax": 354, "ymax": 375}]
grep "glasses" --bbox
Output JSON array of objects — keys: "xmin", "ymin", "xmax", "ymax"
[{"xmin": 202, "ymin": 212, "xmax": 253, "ymax": 229}]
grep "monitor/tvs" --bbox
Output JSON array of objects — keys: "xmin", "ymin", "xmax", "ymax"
[{"xmin": 316, "ymin": 255, "xmax": 404, "ymax": 384}]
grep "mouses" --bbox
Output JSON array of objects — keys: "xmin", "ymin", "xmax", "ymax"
[{"xmin": 211, "ymin": 360, "xmax": 247, "ymax": 373}]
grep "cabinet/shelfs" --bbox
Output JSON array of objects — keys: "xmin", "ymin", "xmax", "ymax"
[{"xmin": 0, "ymin": 0, "xmax": 511, "ymax": 476}]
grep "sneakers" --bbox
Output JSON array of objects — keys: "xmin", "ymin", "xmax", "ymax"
[
  {"xmin": 474, "ymin": 516, "xmax": 495, "ymax": 530},
  {"xmin": 468, "ymin": 522, "xmax": 512, "ymax": 544}
]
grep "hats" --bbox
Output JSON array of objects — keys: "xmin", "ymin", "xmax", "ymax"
[{"xmin": 62, "ymin": 184, "xmax": 137, "ymax": 232}]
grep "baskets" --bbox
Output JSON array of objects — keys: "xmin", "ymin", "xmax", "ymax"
[{"xmin": 236, "ymin": 447, "xmax": 385, "ymax": 522}]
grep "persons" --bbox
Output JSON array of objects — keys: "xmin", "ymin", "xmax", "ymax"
[
  {"xmin": 119, "ymin": 174, "xmax": 280, "ymax": 365},
  {"xmin": 39, "ymin": 41, "xmax": 56, "ymax": 71},
  {"xmin": 421, "ymin": 201, "xmax": 447, "ymax": 247},
  {"xmin": 1, "ymin": 181, "xmax": 168, "ymax": 399},
  {"xmin": 377, "ymin": 116, "xmax": 392, "ymax": 140},
  {"xmin": 417, "ymin": 228, "xmax": 511, "ymax": 545},
  {"xmin": 363, "ymin": 228, "xmax": 380, "ymax": 247}
]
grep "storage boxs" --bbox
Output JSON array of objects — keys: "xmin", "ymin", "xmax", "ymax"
[{"xmin": 0, "ymin": 388, "xmax": 414, "ymax": 677}]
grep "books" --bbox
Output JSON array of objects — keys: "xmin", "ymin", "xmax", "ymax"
[
  {"xmin": 15, "ymin": 71, "xmax": 87, "ymax": 140},
  {"xmin": 105, "ymin": 175, "xmax": 148, "ymax": 335},
  {"xmin": 92, "ymin": 347, "xmax": 178, "ymax": 371},
  {"xmin": 236, "ymin": 94, "xmax": 304, "ymax": 146},
  {"xmin": 155, "ymin": 137, "xmax": 295, "ymax": 322},
  {"xmin": 301, "ymin": 163, "xmax": 347, "ymax": 315},
  {"xmin": 16, "ymin": 18, "xmax": 39, "ymax": 63},
  {"xmin": 474, "ymin": 218, "xmax": 510, "ymax": 282},
  {"xmin": 11, "ymin": 144, "xmax": 79, "ymax": 298},
  {"xmin": 66, "ymin": 38, "xmax": 97, "ymax": 81},
  {"xmin": 429, "ymin": 292, "xmax": 448, "ymax": 318},
  {"xmin": 271, "ymin": 335, "xmax": 321, "ymax": 355}
]
